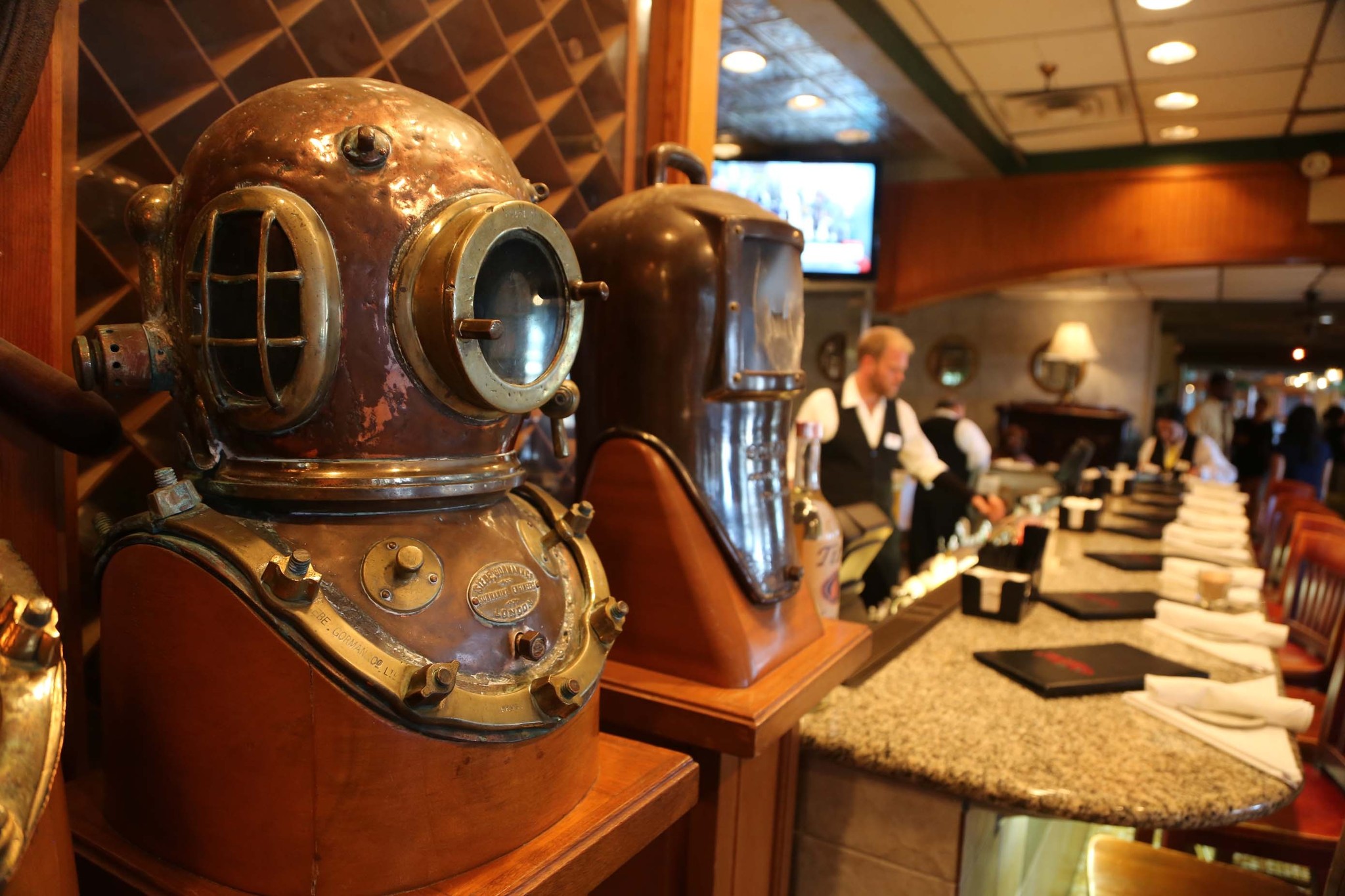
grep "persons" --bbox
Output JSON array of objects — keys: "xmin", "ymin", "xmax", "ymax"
[
  {"xmin": 1138, "ymin": 403, "xmax": 1239, "ymax": 484},
  {"xmin": 1322, "ymin": 406, "xmax": 1345, "ymax": 464},
  {"xmin": 1266, "ymin": 406, "xmax": 1332, "ymax": 505},
  {"xmin": 906, "ymin": 398, "xmax": 991, "ymax": 574},
  {"xmin": 1235, "ymin": 398, "xmax": 1270, "ymax": 477},
  {"xmin": 1186, "ymin": 371, "xmax": 1234, "ymax": 459},
  {"xmin": 788, "ymin": 325, "xmax": 1005, "ymax": 612}
]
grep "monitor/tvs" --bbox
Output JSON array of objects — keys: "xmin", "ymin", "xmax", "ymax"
[{"xmin": 713, "ymin": 146, "xmax": 880, "ymax": 282}]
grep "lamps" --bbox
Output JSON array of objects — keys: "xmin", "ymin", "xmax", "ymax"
[{"xmin": 1044, "ymin": 322, "xmax": 1101, "ymax": 405}]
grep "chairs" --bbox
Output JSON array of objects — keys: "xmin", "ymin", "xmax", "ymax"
[{"xmin": 1137, "ymin": 483, "xmax": 1345, "ymax": 894}]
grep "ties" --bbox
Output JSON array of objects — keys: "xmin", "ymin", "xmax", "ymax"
[{"xmin": 1221, "ymin": 405, "xmax": 1228, "ymax": 457}]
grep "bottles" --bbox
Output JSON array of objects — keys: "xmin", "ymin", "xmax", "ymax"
[{"xmin": 791, "ymin": 417, "xmax": 842, "ymax": 620}]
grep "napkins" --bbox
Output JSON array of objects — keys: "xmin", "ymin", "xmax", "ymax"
[{"xmin": 1123, "ymin": 480, "xmax": 1315, "ymax": 793}]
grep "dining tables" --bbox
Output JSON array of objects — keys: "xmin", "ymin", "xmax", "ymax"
[{"xmin": 790, "ymin": 470, "xmax": 1306, "ymax": 895}]
